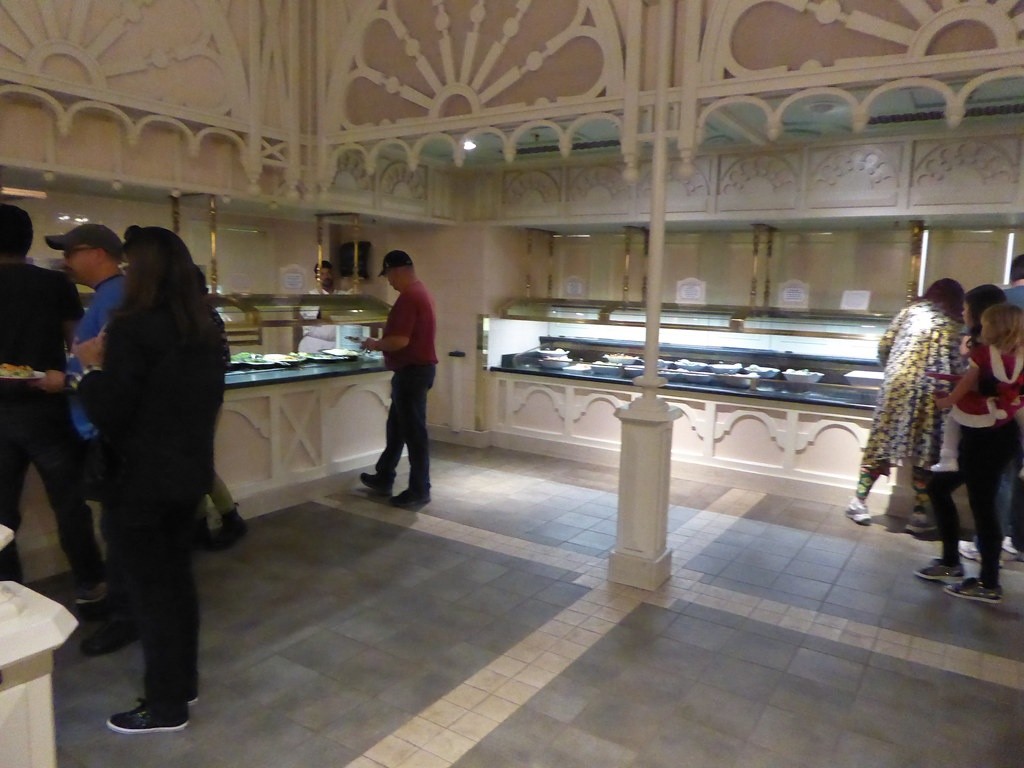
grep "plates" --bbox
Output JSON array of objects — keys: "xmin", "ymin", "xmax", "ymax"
[
  {"xmin": 0, "ymin": 370, "xmax": 45, "ymax": 380},
  {"xmin": 230, "ymin": 347, "xmax": 361, "ymax": 367}
]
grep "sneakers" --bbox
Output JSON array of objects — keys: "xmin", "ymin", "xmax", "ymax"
[
  {"xmin": 943, "ymin": 577, "xmax": 1004, "ymax": 603},
  {"xmin": 845, "ymin": 498, "xmax": 872, "ymax": 523},
  {"xmin": 137, "ymin": 685, "xmax": 199, "ymax": 702},
  {"xmin": 913, "ymin": 558, "xmax": 965, "ymax": 582},
  {"xmin": 107, "ymin": 700, "xmax": 189, "ymax": 735},
  {"xmin": 905, "ymin": 513, "xmax": 938, "ymax": 534},
  {"xmin": 1002, "ymin": 536, "xmax": 1018, "ymax": 554},
  {"xmin": 930, "ymin": 463, "xmax": 959, "ymax": 472},
  {"xmin": 958, "ymin": 540, "xmax": 1004, "ymax": 569}
]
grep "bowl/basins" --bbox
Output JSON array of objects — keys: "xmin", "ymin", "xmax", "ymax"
[
  {"xmin": 782, "ymin": 371, "xmax": 825, "ymax": 383},
  {"xmin": 843, "ymin": 369, "xmax": 884, "ymax": 387},
  {"xmin": 657, "ymin": 359, "xmax": 760, "ymax": 388},
  {"xmin": 538, "ymin": 358, "xmax": 573, "ymax": 369},
  {"xmin": 537, "ymin": 350, "xmax": 570, "ymax": 358},
  {"xmin": 590, "ymin": 355, "xmax": 644, "ymax": 377},
  {"xmin": 744, "ymin": 366, "xmax": 781, "ymax": 380}
]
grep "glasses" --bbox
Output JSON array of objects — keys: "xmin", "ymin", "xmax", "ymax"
[{"xmin": 63, "ymin": 247, "xmax": 91, "ymax": 259}]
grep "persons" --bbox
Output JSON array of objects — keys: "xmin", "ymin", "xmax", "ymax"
[
  {"xmin": 1, "ymin": 207, "xmax": 250, "ymax": 735},
  {"xmin": 313, "ymin": 261, "xmax": 334, "ymax": 295},
  {"xmin": 846, "ymin": 252, "xmax": 1024, "ymax": 605},
  {"xmin": 359, "ymin": 249, "xmax": 438, "ymax": 506}
]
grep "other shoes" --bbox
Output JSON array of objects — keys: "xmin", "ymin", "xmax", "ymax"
[
  {"xmin": 390, "ymin": 482, "xmax": 431, "ymax": 507},
  {"xmin": 75, "ymin": 579, "xmax": 109, "ymax": 604},
  {"xmin": 81, "ymin": 621, "xmax": 140, "ymax": 657},
  {"xmin": 359, "ymin": 471, "xmax": 396, "ymax": 495}
]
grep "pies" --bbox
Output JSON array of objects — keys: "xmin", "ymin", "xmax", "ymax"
[{"xmin": 0, "ymin": 363, "xmax": 35, "ymax": 371}]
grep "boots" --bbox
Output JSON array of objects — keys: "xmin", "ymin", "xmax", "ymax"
[
  {"xmin": 213, "ymin": 509, "xmax": 248, "ymax": 547},
  {"xmin": 193, "ymin": 518, "xmax": 212, "ymax": 550}
]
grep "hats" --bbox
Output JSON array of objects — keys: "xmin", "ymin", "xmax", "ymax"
[
  {"xmin": 378, "ymin": 250, "xmax": 414, "ymax": 275},
  {"xmin": 46, "ymin": 223, "xmax": 123, "ymax": 258}
]
